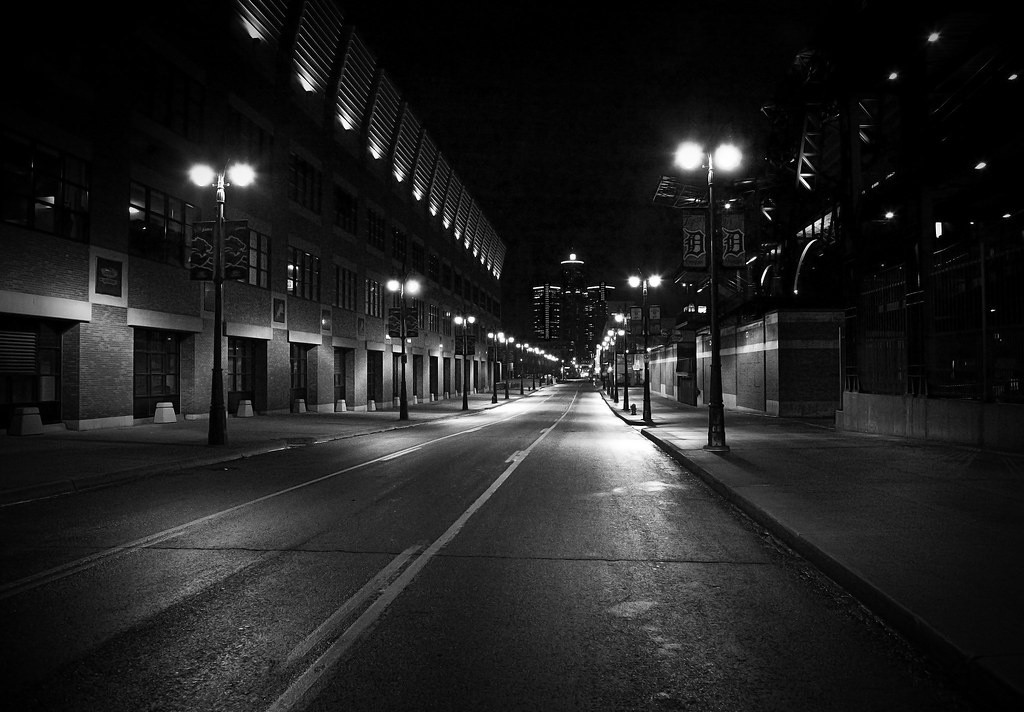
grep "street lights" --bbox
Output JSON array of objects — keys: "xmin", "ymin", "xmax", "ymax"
[
  {"xmin": 187, "ymin": 155, "xmax": 259, "ymax": 445},
  {"xmin": 672, "ymin": 138, "xmax": 744, "ymax": 453},
  {"xmin": 487, "ymin": 330, "xmax": 505, "ymax": 403},
  {"xmin": 386, "ymin": 274, "xmax": 421, "ymax": 423},
  {"xmin": 594, "ymin": 308, "xmax": 632, "ymax": 412},
  {"xmin": 499, "ymin": 337, "xmax": 559, "ymax": 399},
  {"xmin": 453, "ymin": 312, "xmax": 476, "ymax": 411},
  {"xmin": 627, "ymin": 272, "xmax": 663, "ymax": 422}
]
